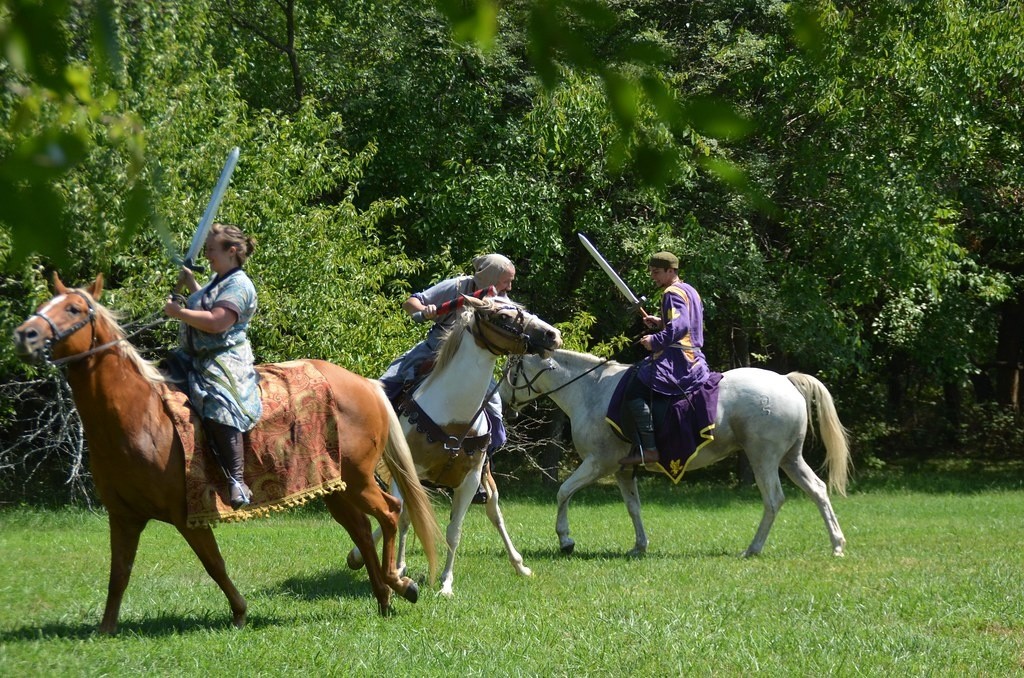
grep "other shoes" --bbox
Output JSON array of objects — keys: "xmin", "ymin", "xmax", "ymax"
[
  {"xmin": 473, "ymin": 483, "xmax": 487, "ymax": 502},
  {"xmin": 617, "ymin": 448, "xmax": 659, "ymax": 464}
]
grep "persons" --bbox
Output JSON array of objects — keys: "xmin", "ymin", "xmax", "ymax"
[
  {"xmin": 166, "ymin": 223, "xmax": 263, "ymax": 510},
  {"xmin": 379, "ymin": 254, "xmax": 515, "ymax": 504},
  {"xmin": 618, "ymin": 252, "xmax": 711, "ymax": 464}
]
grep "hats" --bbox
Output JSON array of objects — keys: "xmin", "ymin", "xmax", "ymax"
[{"xmin": 649, "ymin": 252, "xmax": 678, "ymax": 269}]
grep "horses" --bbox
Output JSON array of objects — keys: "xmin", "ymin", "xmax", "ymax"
[
  {"xmin": 13, "ymin": 271, "xmax": 441, "ymax": 636},
  {"xmin": 348, "ymin": 294, "xmax": 563, "ymax": 598},
  {"xmin": 500, "ymin": 347, "xmax": 852, "ymax": 559}
]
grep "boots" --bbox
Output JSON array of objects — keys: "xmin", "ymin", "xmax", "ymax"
[{"xmin": 209, "ymin": 420, "xmax": 253, "ymax": 511}]
